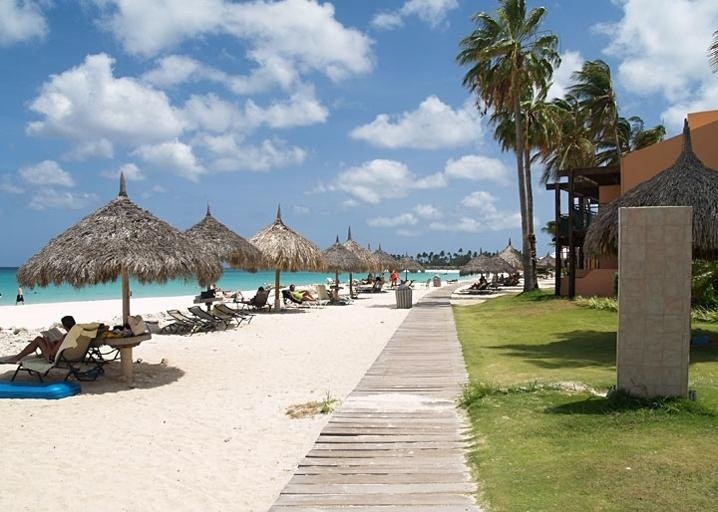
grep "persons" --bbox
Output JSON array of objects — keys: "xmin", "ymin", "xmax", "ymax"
[
  {"xmin": 4, "ymin": 314, "xmax": 77, "ymax": 364},
  {"xmin": 222, "ymin": 290, "xmax": 245, "ymax": 301},
  {"xmin": 15, "ymin": 285, "xmax": 24, "ymax": 306},
  {"xmin": 352, "ymin": 270, "xmax": 399, "ymax": 292},
  {"xmin": 287, "ymin": 284, "xmax": 318, "ymax": 303},
  {"xmin": 467, "ymin": 272, "xmax": 520, "ymax": 290},
  {"xmin": 240, "ymin": 285, "xmax": 266, "ymax": 305}
]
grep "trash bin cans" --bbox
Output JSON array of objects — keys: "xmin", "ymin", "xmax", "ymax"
[
  {"xmin": 395, "ymin": 284, "xmax": 412, "ymax": 309},
  {"xmin": 433, "ymin": 276, "xmax": 441, "ymax": 287}
]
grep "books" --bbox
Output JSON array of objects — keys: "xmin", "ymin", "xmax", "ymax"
[{"xmin": 39, "ymin": 327, "xmax": 63, "ymax": 344}]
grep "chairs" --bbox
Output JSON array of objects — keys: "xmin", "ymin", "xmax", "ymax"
[
  {"xmin": 161, "ymin": 302, "xmax": 276, "ymax": 335},
  {"xmin": 461, "ymin": 275, "xmax": 520, "ymax": 293},
  {"xmin": 282, "ymin": 290, "xmax": 330, "ymax": 308},
  {"xmin": 10, "ymin": 322, "xmax": 103, "ymax": 384},
  {"xmin": 234, "ymin": 290, "xmax": 272, "ymax": 311},
  {"xmin": 391, "ymin": 280, "xmax": 414, "ymax": 290},
  {"xmin": 355, "ymin": 280, "xmax": 380, "ymax": 293}
]
